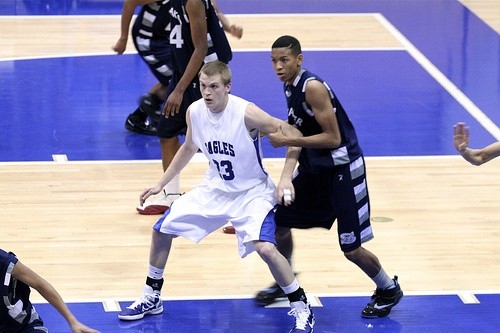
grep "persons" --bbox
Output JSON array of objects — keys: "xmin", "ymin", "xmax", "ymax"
[
  {"xmin": 452, "ymin": 122, "xmax": 500, "ymax": 166},
  {"xmin": 252, "ymin": 35, "xmax": 403, "ymax": 317},
  {"xmin": 0, "ymin": 249, "xmax": 102, "ymax": 333},
  {"xmin": 111, "ymin": 0, "xmax": 243, "ymax": 234},
  {"xmin": 119, "ymin": 61, "xmax": 316, "ymax": 333}
]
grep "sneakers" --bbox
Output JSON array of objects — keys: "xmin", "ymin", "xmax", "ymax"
[
  {"xmin": 118, "ymin": 284, "xmax": 163, "ymax": 320},
  {"xmin": 223, "ymin": 225, "xmax": 235, "ymax": 233},
  {"xmin": 136, "ymin": 189, "xmax": 186, "ymax": 215},
  {"xmin": 287, "ymin": 301, "xmax": 315, "ymax": 333},
  {"xmin": 361, "ymin": 275, "xmax": 403, "ymax": 318},
  {"xmin": 125, "ymin": 112, "xmax": 157, "ymax": 136},
  {"xmin": 254, "ymin": 272, "xmax": 297, "ymax": 305},
  {"xmin": 138, "ymin": 92, "xmax": 162, "ymax": 127}
]
